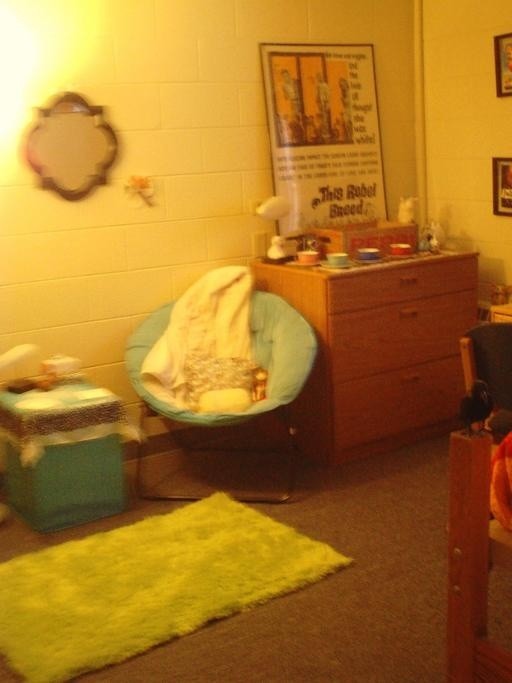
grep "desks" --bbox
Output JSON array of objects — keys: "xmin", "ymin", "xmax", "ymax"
[{"xmin": 446, "ymin": 411, "xmax": 511, "ymax": 683}]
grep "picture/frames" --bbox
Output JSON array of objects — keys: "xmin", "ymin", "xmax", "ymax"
[
  {"xmin": 259, "ymin": 42, "xmax": 389, "ymax": 242},
  {"xmin": 494, "ymin": 32, "xmax": 512, "ymax": 97},
  {"xmin": 492, "ymin": 157, "xmax": 512, "ymax": 217}
]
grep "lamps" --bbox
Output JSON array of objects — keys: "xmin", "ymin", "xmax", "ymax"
[{"xmin": 255, "ymin": 196, "xmax": 297, "ymax": 260}]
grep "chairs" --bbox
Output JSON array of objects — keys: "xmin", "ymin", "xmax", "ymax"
[
  {"xmin": 124, "ymin": 289, "xmax": 318, "ymax": 502},
  {"xmin": 460, "ymin": 321, "xmax": 512, "ymax": 422}
]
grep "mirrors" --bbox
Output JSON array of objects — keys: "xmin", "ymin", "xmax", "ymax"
[{"xmin": 25, "ymin": 91, "xmax": 118, "ymax": 202}]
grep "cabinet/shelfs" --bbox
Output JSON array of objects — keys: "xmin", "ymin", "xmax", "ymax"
[
  {"xmin": 251, "ymin": 248, "xmax": 481, "ymax": 472},
  {"xmin": 491, "ymin": 303, "xmax": 512, "ymax": 323}
]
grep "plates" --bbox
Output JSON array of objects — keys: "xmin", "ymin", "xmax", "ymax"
[
  {"xmin": 320, "ymin": 265, "xmax": 352, "ymax": 273},
  {"xmin": 386, "ymin": 254, "xmax": 416, "ymax": 260},
  {"xmin": 287, "ymin": 261, "xmax": 320, "ymax": 266},
  {"xmin": 352, "ymin": 258, "xmax": 382, "ymax": 265}
]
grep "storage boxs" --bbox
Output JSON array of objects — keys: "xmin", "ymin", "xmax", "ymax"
[{"xmin": 304, "ymin": 222, "xmax": 418, "ymax": 255}]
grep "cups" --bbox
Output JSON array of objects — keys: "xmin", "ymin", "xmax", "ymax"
[
  {"xmin": 390, "ymin": 244, "xmax": 410, "ymax": 255},
  {"xmin": 327, "ymin": 252, "xmax": 348, "ymax": 264},
  {"xmin": 358, "ymin": 248, "xmax": 379, "ymax": 259},
  {"xmin": 298, "ymin": 251, "xmax": 319, "ymax": 262}
]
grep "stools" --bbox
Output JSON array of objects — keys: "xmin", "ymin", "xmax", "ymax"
[{"xmin": 0, "ymin": 379, "xmax": 126, "ymax": 532}]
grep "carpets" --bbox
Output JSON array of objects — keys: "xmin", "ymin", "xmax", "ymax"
[{"xmin": 0, "ymin": 491, "xmax": 354, "ymax": 682}]
